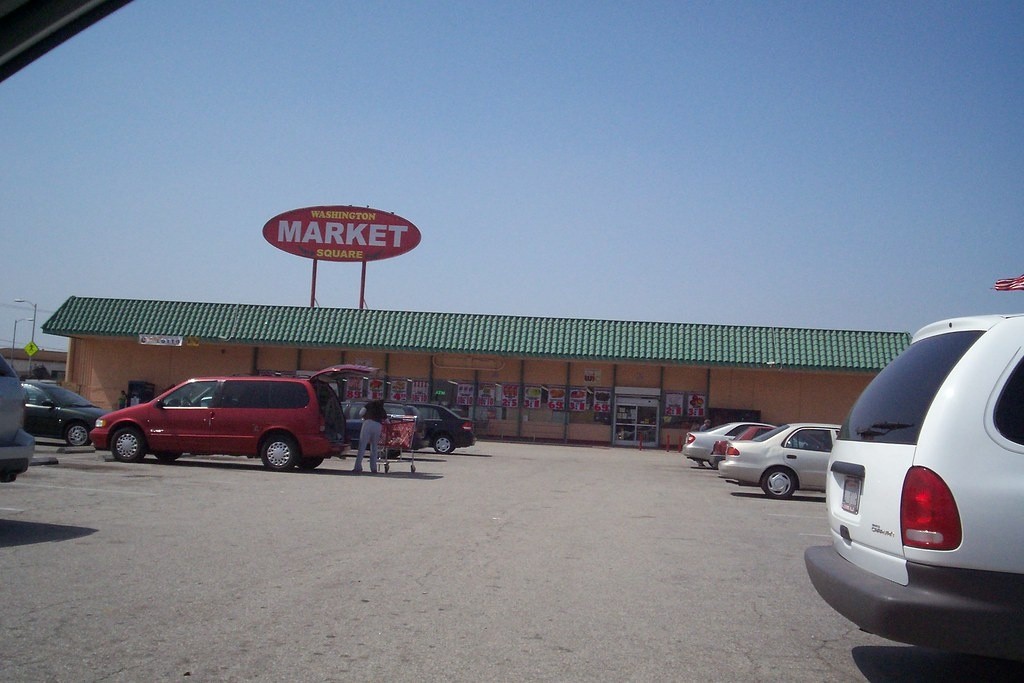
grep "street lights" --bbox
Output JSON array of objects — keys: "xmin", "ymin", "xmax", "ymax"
[
  {"xmin": 14, "ymin": 298, "xmax": 37, "ymax": 377},
  {"xmin": 9, "ymin": 318, "xmax": 34, "ymax": 368}
]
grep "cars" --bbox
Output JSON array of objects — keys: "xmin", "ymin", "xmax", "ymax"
[
  {"xmin": 681, "ymin": 422, "xmax": 778, "ymax": 469},
  {"xmin": 717, "ymin": 423, "xmax": 842, "ymax": 501},
  {"xmin": 20, "ymin": 380, "xmax": 113, "ymax": 448},
  {"xmin": 404, "ymin": 401, "xmax": 478, "ymax": 456},
  {"xmin": 0, "ymin": 351, "xmax": 37, "ymax": 483},
  {"xmin": 709, "ymin": 425, "xmax": 829, "ymax": 463},
  {"xmin": 338, "ymin": 398, "xmax": 424, "ymax": 461}
]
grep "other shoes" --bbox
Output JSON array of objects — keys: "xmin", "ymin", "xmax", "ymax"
[
  {"xmin": 352, "ymin": 469, "xmax": 361, "ymax": 473},
  {"xmin": 699, "ymin": 463, "xmax": 705, "ymax": 467},
  {"xmin": 372, "ymin": 470, "xmax": 377, "ymax": 474}
]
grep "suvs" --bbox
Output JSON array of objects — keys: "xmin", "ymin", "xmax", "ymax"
[
  {"xmin": 88, "ymin": 364, "xmax": 382, "ymax": 472},
  {"xmin": 804, "ymin": 314, "xmax": 1024, "ymax": 664}
]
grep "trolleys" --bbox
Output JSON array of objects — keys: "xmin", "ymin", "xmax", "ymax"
[{"xmin": 379, "ymin": 413, "xmax": 418, "ymax": 474}]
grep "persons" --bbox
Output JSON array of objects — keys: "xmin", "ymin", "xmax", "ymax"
[
  {"xmin": 117, "ymin": 390, "xmax": 126, "ymax": 410},
  {"xmin": 351, "ymin": 398, "xmax": 390, "ymax": 474}
]
